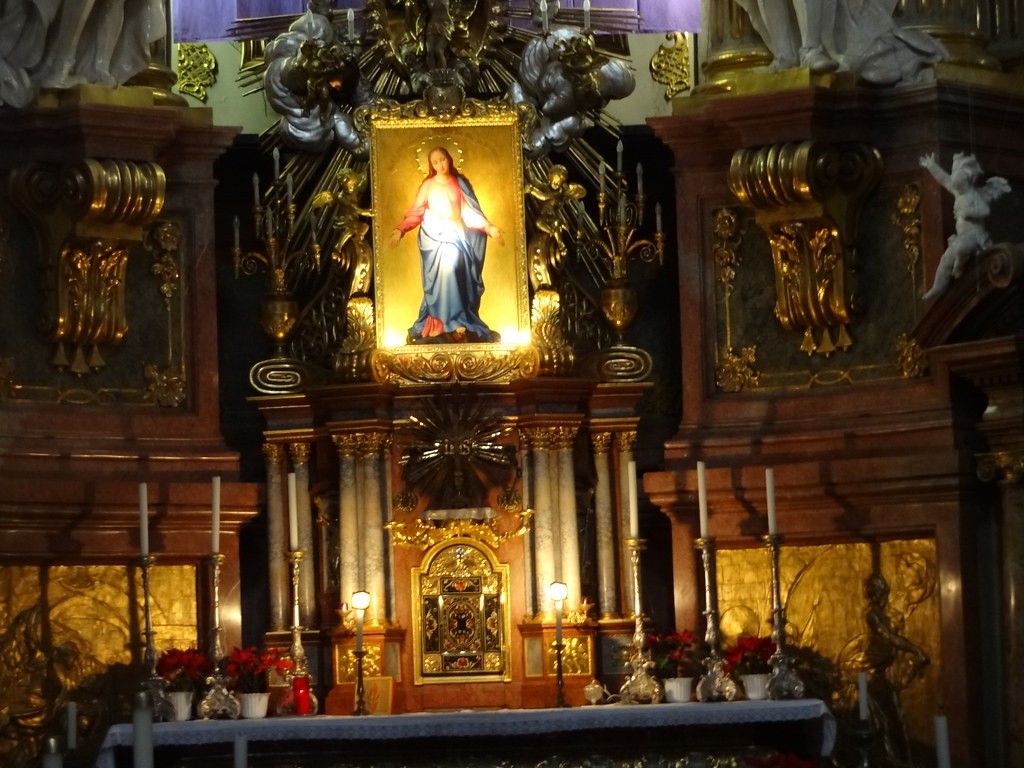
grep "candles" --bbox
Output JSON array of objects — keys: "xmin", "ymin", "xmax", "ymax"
[
  {"xmin": 550, "ymin": 599, "xmax": 569, "ymax": 642},
  {"xmin": 351, "ymin": 609, "xmax": 371, "ymax": 651},
  {"xmin": 765, "ymin": 469, "xmax": 778, "ymax": 534},
  {"xmin": 233, "ymin": 733, "xmax": 248, "ymax": 767},
  {"xmin": 138, "ymin": 482, "xmax": 150, "ymax": 556},
  {"xmin": 627, "ymin": 460, "xmax": 639, "ymax": 538},
  {"xmin": 287, "ymin": 472, "xmax": 300, "ymax": 550},
  {"xmin": 132, "ymin": 706, "xmax": 153, "ymax": 767},
  {"xmin": 66, "ymin": 699, "xmax": 77, "ymax": 749},
  {"xmin": 697, "ymin": 462, "xmax": 710, "ymax": 537},
  {"xmin": 211, "ymin": 476, "xmax": 221, "ymax": 553},
  {"xmin": 933, "ymin": 714, "xmax": 952, "ymax": 767},
  {"xmin": 858, "ymin": 671, "xmax": 868, "ymax": 719}
]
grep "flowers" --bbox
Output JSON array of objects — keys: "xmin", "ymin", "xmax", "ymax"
[
  {"xmin": 154, "ymin": 647, "xmax": 206, "ymax": 689},
  {"xmin": 650, "ymin": 631, "xmax": 701, "ymax": 674},
  {"xmin": 723, "ymin": 631, "xmax": 778, "ymax": 671},
  {"xmin": 218, "ymin": 644, "xmax": 295, "ymax": 693}
]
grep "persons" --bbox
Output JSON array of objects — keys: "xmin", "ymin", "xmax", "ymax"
[
  {"xmin": 919, "ymin": 151, "xmax": 990, "ymax": 300},
  {"xmin": 0, "ymin": 0, "xmax": 178, "ymax": 108},
  {"xmin": 736, "ymin": 0, "xmax": 949, "ymax": 83}
]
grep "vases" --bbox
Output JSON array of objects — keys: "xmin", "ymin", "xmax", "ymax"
[
  {"xmin": 663, "ymin": 676, "xmax": 693, "ymax": 701},
  {"xmin": 736, "ymin": 673, "xmax": 771, "ymax": 698},
  {"xmin": 163, "ymin": 691, "xmax": 193, "ymax": 720},
  {"xmin": 236, "ymin": 692, "xmax": 270, "ymax": 718}
]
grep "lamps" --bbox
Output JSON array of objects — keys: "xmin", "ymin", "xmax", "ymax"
[
  {"xmin": 574, "ymin": 140, "xmax": 668, "ymax": 348},
  {"xmin": 231, "ymin": 148, "xmax": 321, "ymax": 355},
  {"xmin": 352, "ymin": 590, "xmax": 370, "ymax": 609},
  {"xmin": 549, "ymin": 582, "xmax": 568, "ymax": 599}
]
